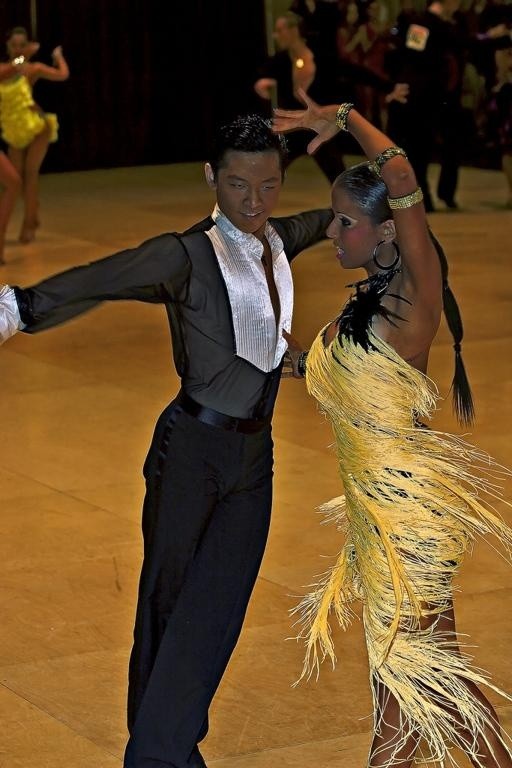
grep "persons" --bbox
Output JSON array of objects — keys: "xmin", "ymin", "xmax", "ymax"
[
  {"xmin": 270, "ymin": 86, "xmax": 512, "ymax": 768},
  {"xmin": 253, "ymin": 1, "xmax": 512, "ymax": 213},
  {"xmin": 2, "ymin": 25, "xmax": 71, "ymax": 242},
  {"xmin": 2, "ymin": 39, "xmax": 38, "ymax": 269},
  {"xmin": 0, "ymin": 112, "xmax": 336, "ymax": 768}
]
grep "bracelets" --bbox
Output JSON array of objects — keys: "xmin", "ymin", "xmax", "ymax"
[
  {"xmin": 299, "ymin": 350, "xmax": 308, "ymax": 377},
  {"xmin": 13, "ymin": 55, "xmax": 27, "ymax": 71},
  {"xmin": 336, "ymin": 100, "xmax": 352, "ymax": 133}
]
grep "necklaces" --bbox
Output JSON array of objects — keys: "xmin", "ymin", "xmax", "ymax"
[{"xmin": 261, "ymin": 254, "xmax": 267, "ymax": 265}]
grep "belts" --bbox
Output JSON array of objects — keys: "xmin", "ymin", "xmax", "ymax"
[{"xmin": 176, "ymin": 390, "xmax": 274, "ymax": 435}]
